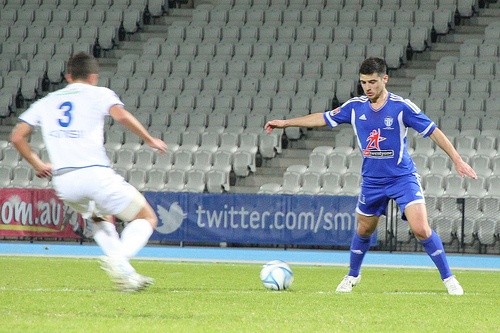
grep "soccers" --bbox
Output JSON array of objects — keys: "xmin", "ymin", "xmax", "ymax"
[{"xmin": 260, "ymin": 260, "xmax": 295, "ymax": 290}]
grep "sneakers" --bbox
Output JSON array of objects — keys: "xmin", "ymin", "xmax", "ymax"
[
  {"xmin": 336, "ymin": 273, "xmax": 362, "ymax": 293},
  {"xmin": 98, "ymin": 255, "xmax": 154, "ymax": 292},
  {"xmin": 442, "ymin": 274, "xmax": 464, "ymax": 297}
]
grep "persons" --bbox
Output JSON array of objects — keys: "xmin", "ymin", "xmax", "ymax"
[
  {"xmin": 12, "ymin": 52, "xmax": 168, "ymax": 292},
  {"xmin": 265, "ymin": 57, "xmax": 479, "ymax": 296}
]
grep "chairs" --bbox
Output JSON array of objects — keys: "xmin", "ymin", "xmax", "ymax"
[{"xmin": 0, "ymin": 0, "xmax": 500, "ymax": 255}]
